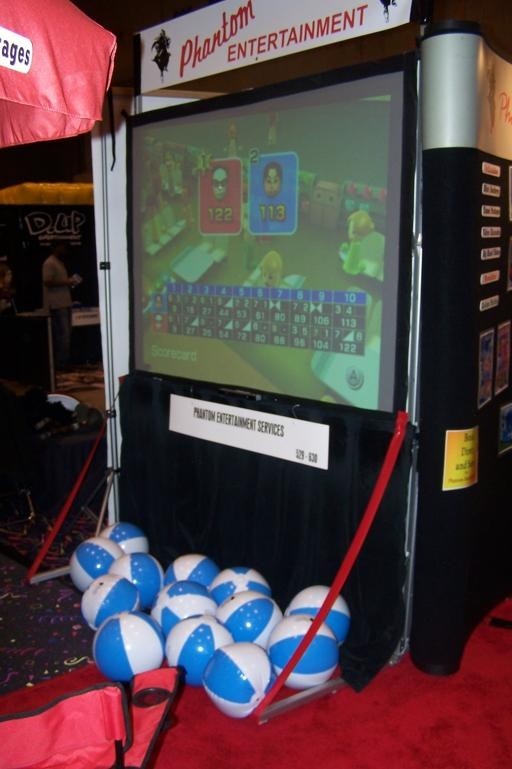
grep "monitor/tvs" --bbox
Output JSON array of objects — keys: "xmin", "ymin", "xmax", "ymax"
[{"xmin": 126, "ymin": 64, "xmax": 413, "ymax": 416}]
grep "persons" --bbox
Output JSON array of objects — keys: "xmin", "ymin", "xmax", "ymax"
[
  {"xmin": 38, "ymin": 238, "xmax": 74, "ymax": 380},
  {"xmin": 0, "ymin": 264, "xmax": 14, "ymax": 320}
]
